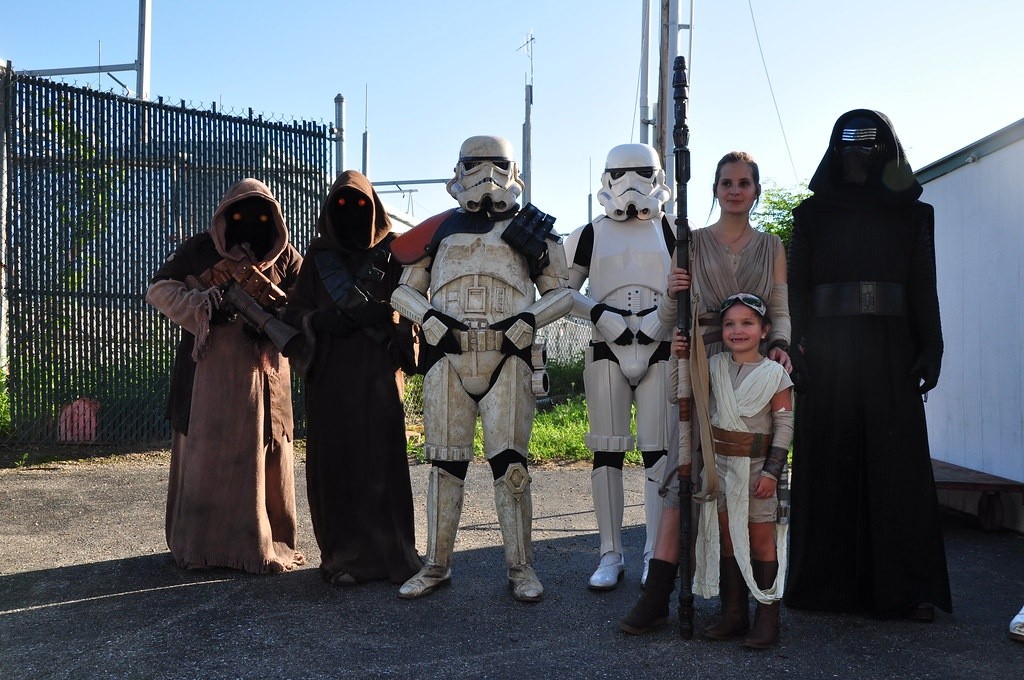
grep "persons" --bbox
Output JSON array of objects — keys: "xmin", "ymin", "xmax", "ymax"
[
  {"xmin": 562, "ymin": 143, "xmax": 678, "ymax": 592},
  {"xmin": 285, "ymin": 171, "xmax": 430, "ymax": 586},
  {"xmin": 616, "ymin": 151, "xmax": 792, "ymax": 633},
  {"xmin": 389, "ymin": 135, "xmax": 574, "ymax": 604},
  {"xmin": 144, "ymin": 177, "xmax": 309, "ymax": 573},
  {"xmin": 790, "ymin": 108, "xmax": 954, "ymax": 623},
  {"xmin": 666, "ymin": 292, "xmax": 796, "ymax": 649}
]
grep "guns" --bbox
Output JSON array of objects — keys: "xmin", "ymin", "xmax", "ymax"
[{"xmin": 184, "ymin": 274, "xmax": 306, "ymax": 359}]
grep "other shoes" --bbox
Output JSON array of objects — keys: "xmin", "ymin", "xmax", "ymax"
[
  {"xmin": 507, "ymin": 566, "xmax": 543, "ymax": 603},
  {"xmin": 905, "ymin": 601, "xmax": 935, "ymax": 621},
  {"xmin": 588, "ymin": 552, "xmax": 624, "ymax": 591},
  {"xmin": 398, "ymin": 567, "xmax": 452, "ymax": 598},
  {"xmin": 337, "ymin": 573, "xmax": 357, "ymax": 586},
  {"xmin": 641, "ymin": 552, "xmax": 653, "ymax": 587}
]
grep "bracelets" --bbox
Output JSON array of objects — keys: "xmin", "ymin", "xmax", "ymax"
[{"xmin": 770, "ymin": 339, "xmax": 791, "ymax": 354}]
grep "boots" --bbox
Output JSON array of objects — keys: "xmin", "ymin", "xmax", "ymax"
[
  {"xmin": 621, "ymin": 558, "xmax": 678, "ymax": 635},
  {"xmin": 741, "ymin": 558, "xmax": 781, "ymax": 649},
  {"xmin": 699, "ymin": 556, "xmax": 750, "ymax": 639}
]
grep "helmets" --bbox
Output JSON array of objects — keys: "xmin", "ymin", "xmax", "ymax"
[
  {"xmin": 596, "ymin": 143, "xmax": 671, "ymax": 222},
  {"xmin": 446, "ymin": 136, "xmax": 525, "ymax": 211}
]
